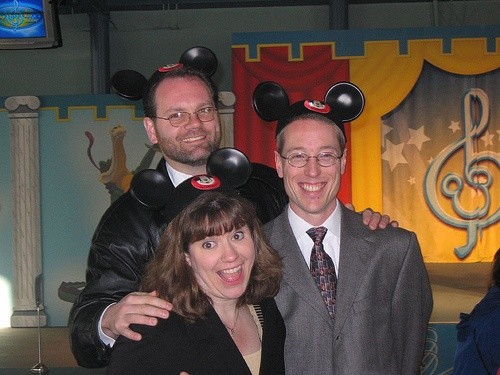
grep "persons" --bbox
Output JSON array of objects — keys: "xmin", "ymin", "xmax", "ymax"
[
  {"xmin": 253, "ymin": 81, "xmax": 433, "ymax": 375},
  {"xmin": 109, "ymin": 148, "xmax": 287, "ymax": 375},
  {"xmin": 69, "ymin": 46, "xmax": 399, "ymax": 369},
  {"xmin": 455, "ymin": 247, "xmax": 500, "ymax": 375}
]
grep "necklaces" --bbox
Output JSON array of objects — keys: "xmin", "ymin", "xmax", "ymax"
[{"xmin": 224, "ymin": 307, "xmax": 240, "ymax": 337}]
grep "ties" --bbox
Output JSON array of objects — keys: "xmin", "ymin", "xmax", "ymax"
[{"xmin": 306, "ymin": 226, "xmax": 337, "ymax": 322}]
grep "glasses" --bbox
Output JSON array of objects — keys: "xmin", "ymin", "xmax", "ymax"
[
  {"xmin": 151, "ymin": 107, "xmax": 218, "ymax": 127},
  {"xmin": 280, "ymin": 153, "xmax": 343, "ymax": 168}
]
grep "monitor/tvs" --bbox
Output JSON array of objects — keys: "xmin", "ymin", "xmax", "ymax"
[{"xmin": 0, "ymin": 0, "xmax": 63, "ymax": 50}]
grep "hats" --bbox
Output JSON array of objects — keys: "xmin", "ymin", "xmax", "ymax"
[
  {"xmin": 132, "ymin": 147, "xmax": 252, "ymax": 225},
  {"xmin": 253, "ymin": 82, "xmax": 365, "ymax": 144},
  {"xmin": 110, "ymin": 46, "xmax": 217, "ymax": 103}
]
